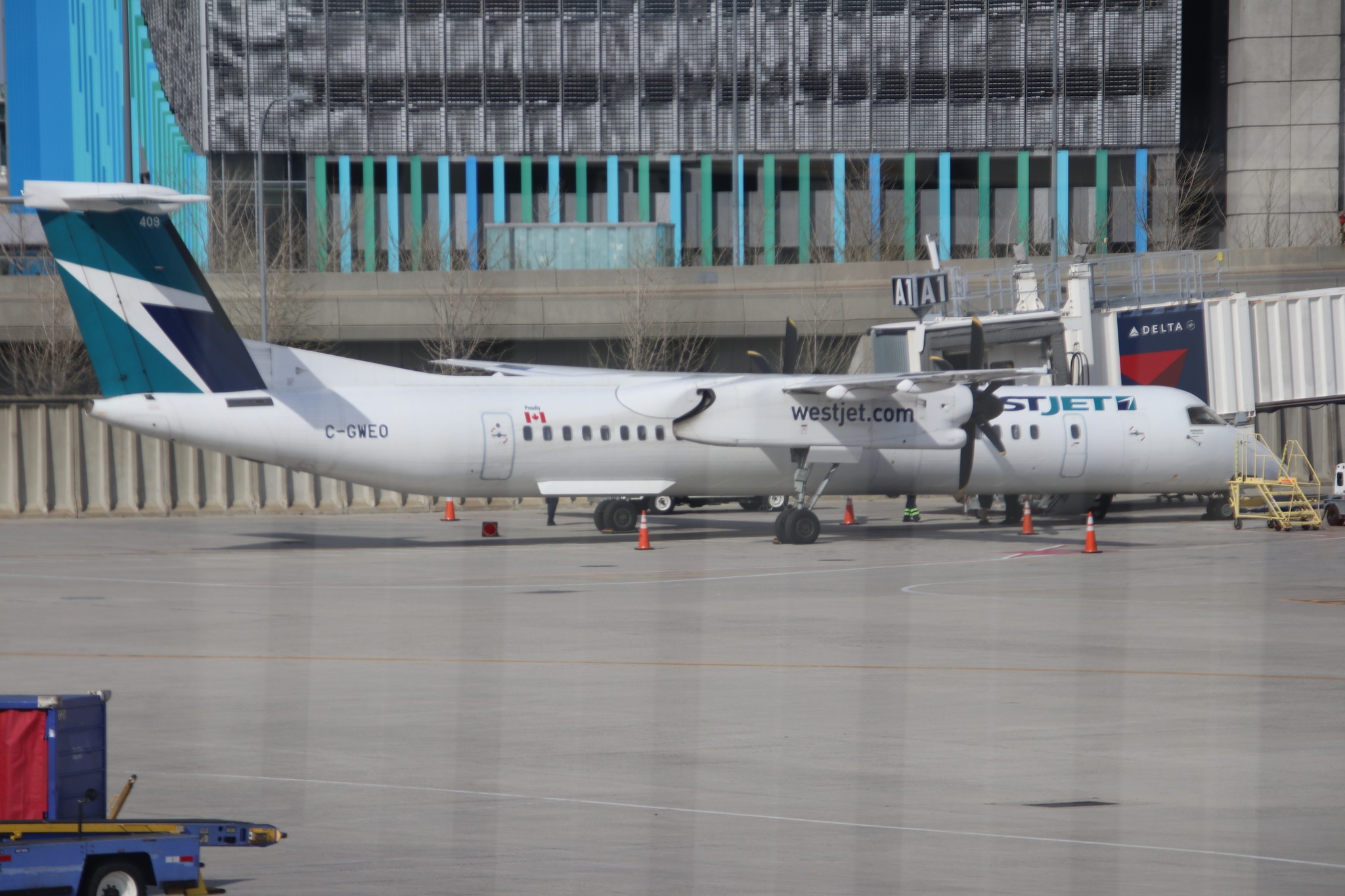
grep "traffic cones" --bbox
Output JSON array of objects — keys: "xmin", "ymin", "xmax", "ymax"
[
  {"xmin": 439, "ymin": 497, "xmax": 460, "ymax": 522},
  {"xmin": 1016, "ymin": 496, "xmax": 1039, "ymax": 535},
  {"xmin": 1080, "ymin": 511, "xmax": 1103, "ymax": 553},
  {"xmin": 838, "ymin": 495, "xmax": 860, "ymax": 526},
  {"xmin": 634, "ymin": 510, "xmax": 654, "ymax": 550},
  {"xmin": 481, "ymin": 521, "xmax": 498, "ymax": 537}
]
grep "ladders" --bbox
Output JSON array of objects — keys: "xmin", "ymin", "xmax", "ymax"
[{"xmin": 1232, "ymin": 431, "xmax": 1326, "ymax": 530}]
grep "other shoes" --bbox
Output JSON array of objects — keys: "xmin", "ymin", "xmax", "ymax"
[
  {"xmin": 979, "ymin": 519, "xmax": 991, "ymax": 525},
  {"xmin": 902, "ymin": 519, "xmax": 913, "ymax": 522},
  {"xmin": 913, "ymin": 517, "xmax": 921, "ymax": 522},
  {"xmin": 999, "ymin": 505, "xmax": 1024, "ymax": 525}
]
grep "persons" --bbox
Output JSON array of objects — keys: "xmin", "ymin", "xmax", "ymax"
[
  {"xmin": 902, "ymin": 495, "xmax": 921, "ymax": 522},
  {"xmin": 977, "ymin": 493, "xmax": 1023, "ymax": 524}
]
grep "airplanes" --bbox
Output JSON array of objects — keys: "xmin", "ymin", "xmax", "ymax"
[{"xmin": 17, "ymin": 174, "xmax": 1297, "ymax": 548}]
